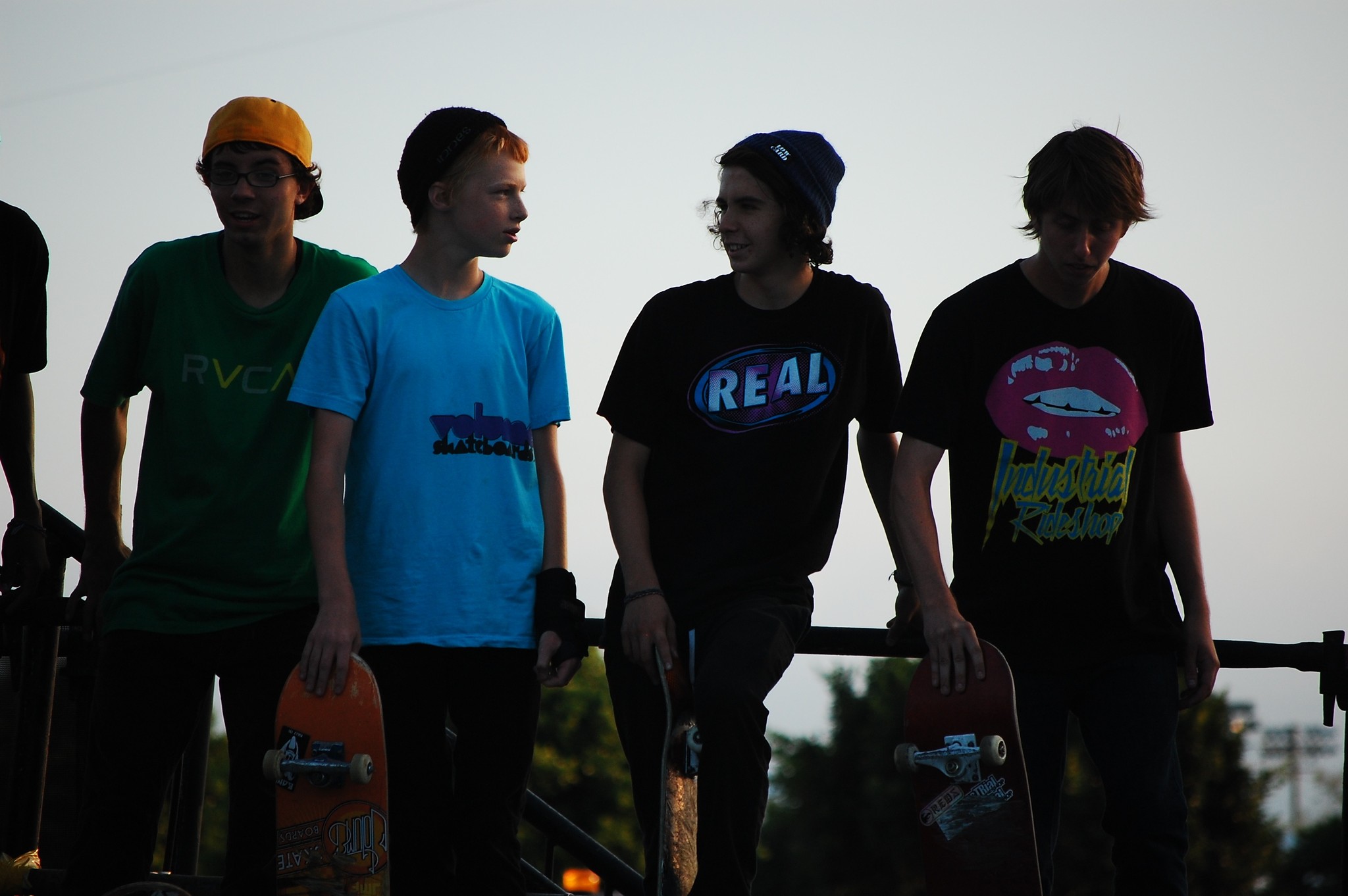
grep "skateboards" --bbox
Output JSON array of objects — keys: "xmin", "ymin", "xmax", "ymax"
[
  {"xmin": 652, "ymin": 641, "xmax": 706, "ymax": 896},
  {"xmin": 262, "ymin": 647, "xmax": 396, "ymax": 896},
  {"xmin": 890, "ymin": 636, "xmax": 1047, "ymax": 894}
]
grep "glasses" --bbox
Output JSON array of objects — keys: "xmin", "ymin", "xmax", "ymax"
[{"xmin": 203, "ymin": 164, "xmax": 298, "ymax": 190}]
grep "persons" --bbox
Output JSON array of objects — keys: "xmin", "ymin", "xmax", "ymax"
[
  {"xmin": 892, "ymin": 126, "xmax": 1222, "ymax": 896},
  {"xmin": 597, "ymin": 128, "xmax": 989, "ymax": 896},
  {"xmin": 77, "ymin": 95, "xmax": 378, "ymax": 896},
  {"xmin": 0, "ymin": 202, "xmax": 52, "ymax": 896},
  {"xmin": 286, "ymin": 106, "xmax": 587, "ymax": 896}
]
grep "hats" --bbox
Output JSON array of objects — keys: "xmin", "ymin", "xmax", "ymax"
[
  {"xmin": 725, "ymin": 128, "xmax": 844, "ymax": 240},
  {"xmin": 208, "ymin": 98, "xmax": 325, "ymax": 220}
]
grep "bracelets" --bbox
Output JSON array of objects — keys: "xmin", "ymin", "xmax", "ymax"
[
  {"xmin": 893, "ymin": 570, "xmax": 914, "ymax": 587},
  {"xmin": 624, "ymin": 587, "xmax": 666, "ymax": 605},
  {"xmin": 7, "ymin": 518, "xmax": 48, "ymax": 538}
]
